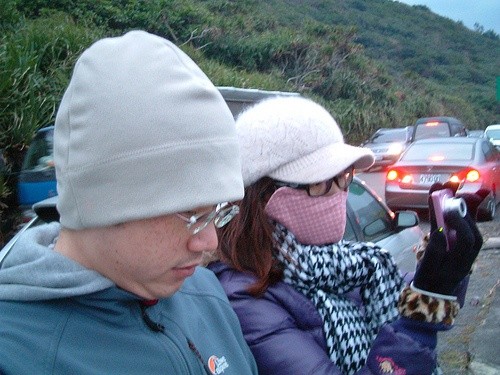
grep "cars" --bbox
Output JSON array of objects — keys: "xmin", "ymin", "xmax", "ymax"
[
  {"xmin": 342, "ymin": 178, "xmax": 428, "ymax": 285},
  {"xmin": 358, "ymin": 127, "xmax": 414, "ymax": 173},
  {"xmin": 483, "ymin": 126, "xmax": 500, "ymax": 147},
  {"xmin": 385, "ymin": 137, "xmax": 499, "ymax": 221}
]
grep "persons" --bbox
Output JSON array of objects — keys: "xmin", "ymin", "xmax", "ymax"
[
  {"xmin": 0, "ymin": 30, "xmax": 257, "ymax": 375},
  {"xmin": 204, "ymin": 97, "xmax": 484, "ymax": 375}
]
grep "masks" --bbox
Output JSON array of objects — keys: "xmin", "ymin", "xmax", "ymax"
[{"xmin": 263, "ymin": 186, "xmax": 350, "ymax": 246}]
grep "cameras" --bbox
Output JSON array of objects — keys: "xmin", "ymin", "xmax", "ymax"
[{"xmin": 428, "ymin": 187, "xmax": 467, "ymax": 252}]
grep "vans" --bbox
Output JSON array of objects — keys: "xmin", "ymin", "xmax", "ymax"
[{"xmin": 409, "ymin": 117, "xmax": 469, "ymax": 146}]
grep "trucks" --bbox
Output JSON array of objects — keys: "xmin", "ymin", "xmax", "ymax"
[{"xmin": 10, "ymin": 83, "xmax": 304, "ymax": 225}]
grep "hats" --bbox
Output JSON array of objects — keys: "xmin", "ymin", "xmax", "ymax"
[
  {"xmin": 52, "ymin": 28, "xmax": 245, "ymax": 230},
  {"xmin": 236, "ymin": 95, "xmax": 375, "ymax": 189}
]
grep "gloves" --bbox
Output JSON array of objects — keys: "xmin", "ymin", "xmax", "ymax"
[{"xmin": 397, "ymin": 180, "xmax": 490, "ymax": 331}]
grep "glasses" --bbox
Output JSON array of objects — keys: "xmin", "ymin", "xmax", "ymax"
[
  {"xmin": 178, "ymin": 202, "xmax": 241, "ymax": 236},
  {"xmin": 270, "ymin": 165, "xmax": 356, "ymax": 198}
]
